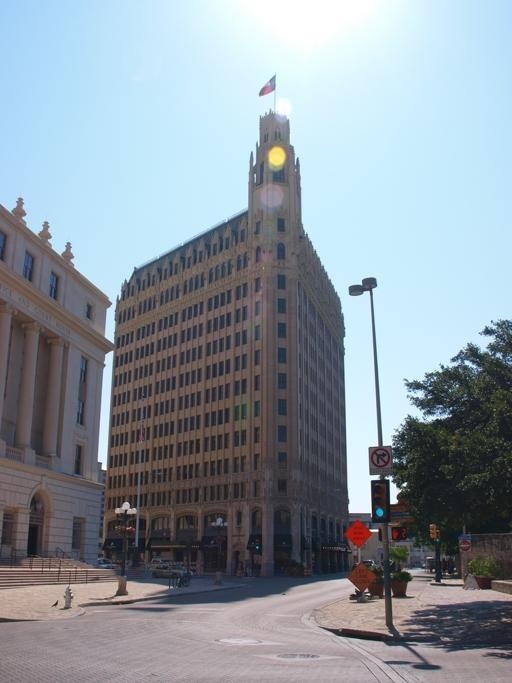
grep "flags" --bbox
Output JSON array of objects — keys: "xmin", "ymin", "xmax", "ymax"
[{"xmin": 259, "ymin": 74, "xmax": 275, "ymax": 96}]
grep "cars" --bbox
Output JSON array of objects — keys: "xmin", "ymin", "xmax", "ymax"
[
  {"xmin": 97, "ymin": 557, "xmax": 121, "ymax": 569},
  {"xmin": 150, "ymin": 563, "xmax": 194, "ymax": 578},
  {"xmin": 352, "ymin": 558, "xmax": 402, "ymax": 574}
]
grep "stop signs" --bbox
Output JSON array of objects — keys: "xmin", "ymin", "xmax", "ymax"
[{"xmin": 459, "ymin": 539, "xmax": 472, "ymax": 552}]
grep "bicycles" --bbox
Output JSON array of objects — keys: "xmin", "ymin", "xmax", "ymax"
[{"xmin": 169, "ymin": 570, "xmax": 192, "ymax": 588}]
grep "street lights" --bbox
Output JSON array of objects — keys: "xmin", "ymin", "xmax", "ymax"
[
  {"xmin": 348, "ymin": 275, "xmax": 395, "ymax": 628},
  {"xmin": 114, "ymin": 500, "xmax": 138, "ymax": 596},
  {"xmin": 210, "ymin": 516, "xmax": 227, "ymax": 585}
]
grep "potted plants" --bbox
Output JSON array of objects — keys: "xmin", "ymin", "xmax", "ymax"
[
  {"xmin": 466, "ymin": 555, "xmax": 503, "ymax": 589},
  {"xmin": 364, "ymin": 563, "xmax": 386, "ymax": 598},
  {"xmin": 390, "ymin": 570, "xmax": 412, "ymax": 596}
]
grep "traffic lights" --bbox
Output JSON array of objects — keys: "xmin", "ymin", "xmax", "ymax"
[
  {"xmin": 369, "ymin": 478, "xmax": 391, "ymax": 523},
  {"xmin": 392, "ymin": 526, "xmax": 408, "ymax": 541},
  {"xmin": 255, "ymin": 534, "xmax": 261, "ymax": 555},
  {"xmin": 429, "ymin": 524, "xmax": 437, "ymax": 539}
]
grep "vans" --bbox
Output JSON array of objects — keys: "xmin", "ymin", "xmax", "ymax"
[{"xmin": 149, "ymin": 558, "xmax": 174, "ymax": 574}]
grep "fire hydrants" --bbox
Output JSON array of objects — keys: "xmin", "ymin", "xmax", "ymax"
[{"xmin": 63, "ymin": 585, "xmax": 74, "ymax": 609}]
